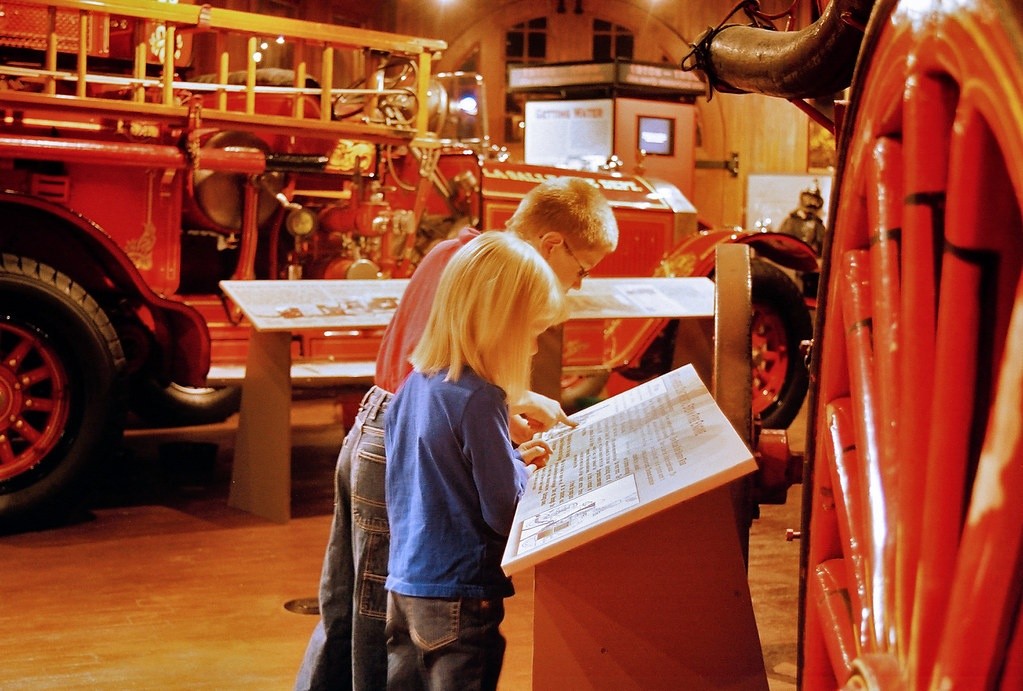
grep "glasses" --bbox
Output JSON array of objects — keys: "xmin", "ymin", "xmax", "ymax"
[{"xmin": 540, "ymin": 233, "xmax": 590, "ymax": 279}]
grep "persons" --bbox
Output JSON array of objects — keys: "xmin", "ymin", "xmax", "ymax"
[
  {"xmin": 382, "ymin": 231, "xmax": 554, "ymax": 691},
  {"xmin": 292, "ymin": 176, "xmax": 619, "ymax": 691}
]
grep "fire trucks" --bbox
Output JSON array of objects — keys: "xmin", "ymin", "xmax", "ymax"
[{"xmin": 0, "ymin": 0, "xmax": 819, "ymax": 536}]
